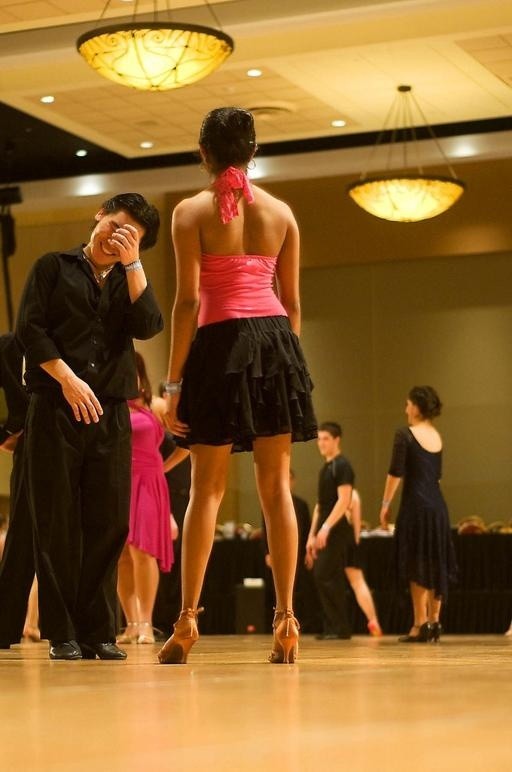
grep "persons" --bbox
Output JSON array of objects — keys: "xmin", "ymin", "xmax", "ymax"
[
  {"xmin": 154, "ymin": 104, "xmax": 324, "ymax": 662},
  {"xmin": 378, "ymin": 386, "xmax": 453, "ymax": 643},
  {"xmin": 0, "ymin": 322, "xmax": 385, "ymax": 652},
  {"xmin": 15, "ymin": 194, "xmax": 165, "ymax": 662}
]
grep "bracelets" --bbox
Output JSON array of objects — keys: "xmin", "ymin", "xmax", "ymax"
[
  {"xmin": 382, "ymin": 501, "xmax": 390, "ymax": 506},
  {"xmin": 123, "ymin": 259, "xmax": 143, "ymax": 273}
]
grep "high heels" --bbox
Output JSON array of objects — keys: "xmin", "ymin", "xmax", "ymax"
[
  {"xmin": 398, "ymin": 622, "xmax": 441, "ymax": 642},
  {"xmin": 268, "ymin": 607, "xmax": 300, "ymax": 663},
  {"xmin": 157, "ymin": 606, "xmax": 204, "ymax": 664}
]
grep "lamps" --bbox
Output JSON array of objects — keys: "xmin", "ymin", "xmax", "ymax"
[
  {"xmin": 347, "ymin": 85, "xmax": 465, "ymax": 222},
  {"xmin": 76, "ymin": 0, "xmax": 235, "ymax": 92}
]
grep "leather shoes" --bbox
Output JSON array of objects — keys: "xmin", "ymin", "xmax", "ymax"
[
  {"xmin": 79, "ymin": 642, "xmax": 127, "ymax": 660},
  {"xmin": 49, "ymin": 640, "xmax": 83, "ymax": 660}
]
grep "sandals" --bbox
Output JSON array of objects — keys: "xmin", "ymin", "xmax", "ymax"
[
  {"xmin": 138, "ymin": 623, "xmax": 155, "ymax": 644},
  {"xmin": 116, "ymin": 622, "xmax": 139, "ymax": 644}
]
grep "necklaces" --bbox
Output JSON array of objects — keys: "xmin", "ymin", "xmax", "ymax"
[{"xmin": 83, "ymin": 256, "xmax": 116, "ymax": 287}]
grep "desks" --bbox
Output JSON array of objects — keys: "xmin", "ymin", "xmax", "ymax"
[{"xmin": 152, "ymin": 533, "xmax": 512, "ymax": 635}]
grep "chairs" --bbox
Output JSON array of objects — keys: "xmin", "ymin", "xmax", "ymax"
[{"xmin": 457, "ymin": 516, "xmax": 512, "ymax": 535}]
